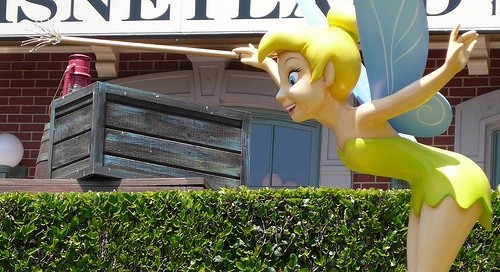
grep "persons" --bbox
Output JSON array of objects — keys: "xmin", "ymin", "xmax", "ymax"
[{"xmin": 233, "ymin": 3, "xmax": 493, "ymax": 272}]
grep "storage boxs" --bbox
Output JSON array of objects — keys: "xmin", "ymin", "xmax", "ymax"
[{"xmin": 49, "ymin": 83, "xmax": 251, "ymax": 190}]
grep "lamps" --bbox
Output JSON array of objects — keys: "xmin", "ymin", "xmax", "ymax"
[{"xmin": 0, "ymin": 132, "xmax": 24, "ymax": 177}]
